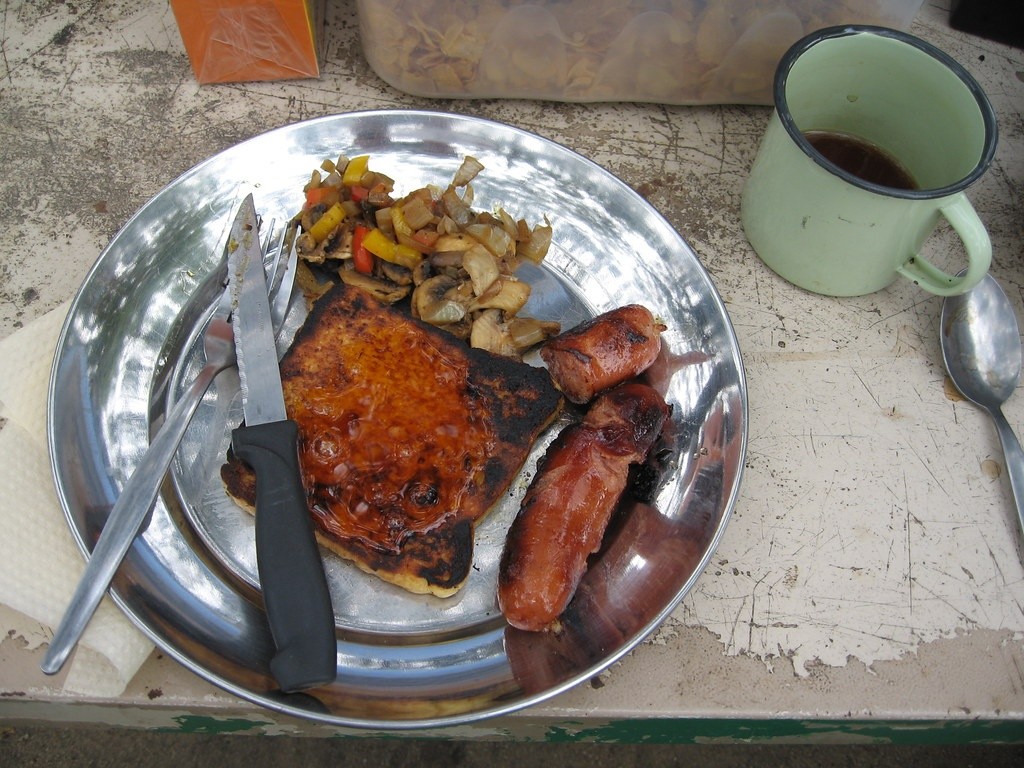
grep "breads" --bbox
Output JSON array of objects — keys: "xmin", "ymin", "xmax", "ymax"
[{"xmin": 221, "ymin": 282, "xmax": 566, "ymax": 598}]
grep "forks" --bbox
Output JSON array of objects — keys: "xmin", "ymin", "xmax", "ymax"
[{"xmin": 40, "ymin": 216, "xmax": 303, "ymax": 674}]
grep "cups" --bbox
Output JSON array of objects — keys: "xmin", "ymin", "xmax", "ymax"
[{"xmin": 740, "ymin": 24, "xmax": 999, "ymax": 300}]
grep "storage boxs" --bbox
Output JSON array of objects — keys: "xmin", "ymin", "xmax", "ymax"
[
  {"xmin": 168, "ymin": 0, "xmax": 324, "ymax": 85},
  {"xmin": 350, "ymin": 0, "xmax": 927, "ymax": 107}
]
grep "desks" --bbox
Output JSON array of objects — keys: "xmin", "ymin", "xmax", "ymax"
[{"xmin": 1, "ymin": 0, "xmax": 1022, "ymax": 768}]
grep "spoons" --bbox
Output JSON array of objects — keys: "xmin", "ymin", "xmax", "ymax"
[{"xmin": 941, "ymin": 267, "xmax": 1023, "ymax": 529}]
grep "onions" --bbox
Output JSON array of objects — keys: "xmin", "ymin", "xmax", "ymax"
[{"xmin": 288, "ymin": 155, "xmax": 557, "ymax": 346}]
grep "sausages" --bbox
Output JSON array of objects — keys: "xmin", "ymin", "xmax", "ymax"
[
  {"xmin": 540, "ymin": 304, "xmax": 669, "ymax": 406},
  {"xmin": 498, "ymin": 382, "xmax": 666, "ymax": 634}
]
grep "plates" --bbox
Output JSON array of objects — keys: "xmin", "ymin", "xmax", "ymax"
[{"xmin": 47, "ymin": 109, "xmax": 749, "ymax": 729}]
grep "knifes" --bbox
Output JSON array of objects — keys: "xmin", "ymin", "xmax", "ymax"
[{"xmin": 227, "ymin": 193, "xmax": 338, "ymax": 693}]
